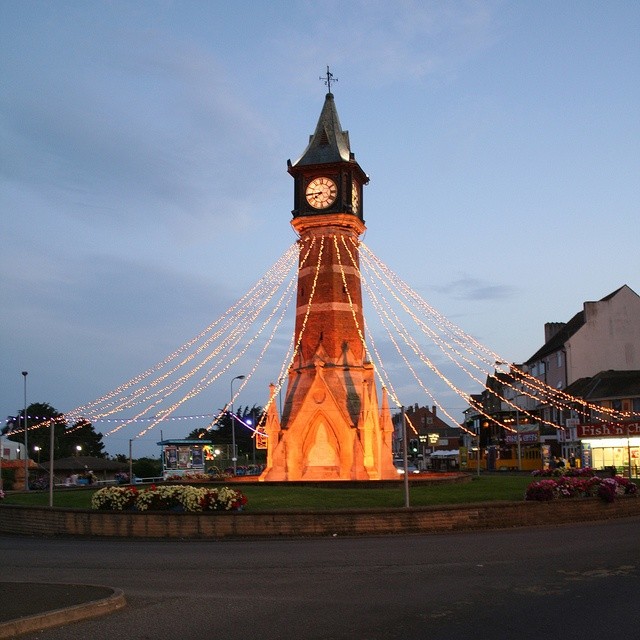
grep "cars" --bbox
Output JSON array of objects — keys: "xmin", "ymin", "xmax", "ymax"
[{"xmin": 394, "ymin": 458, "xmax": 420, "ymax": 476}]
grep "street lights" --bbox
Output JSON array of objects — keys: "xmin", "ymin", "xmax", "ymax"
[
  {"xmin": 33, "ymin": 446, "xmax": 43, "ymax": 481},
  {"xmin": 230, "ymin": 375, "xmax": 246, "ymax": 476},
  {"xmin": 494, "ymin": 359, "xmax": 521, "ymax": 472},
  {"xmin": 21, "ymin": 371, "xmax": 30, "ymax": 492}
]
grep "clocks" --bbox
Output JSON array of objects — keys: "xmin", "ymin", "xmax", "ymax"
[
  {"xmin": 351, "ymin": 180, "xmax": 361, "ymax": 215},
  {"xmin": 304, "ymin": 175, "xmax": 340, "ymax": 211}
]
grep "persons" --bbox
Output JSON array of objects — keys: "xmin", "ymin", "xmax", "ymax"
[
  {"xmin": 568, "ymin": 452, "xmax": 577, "ymax": 468},
  {"xmin": 548, "ymin": 455, "xmax": 567, "ymax": 469}
]
